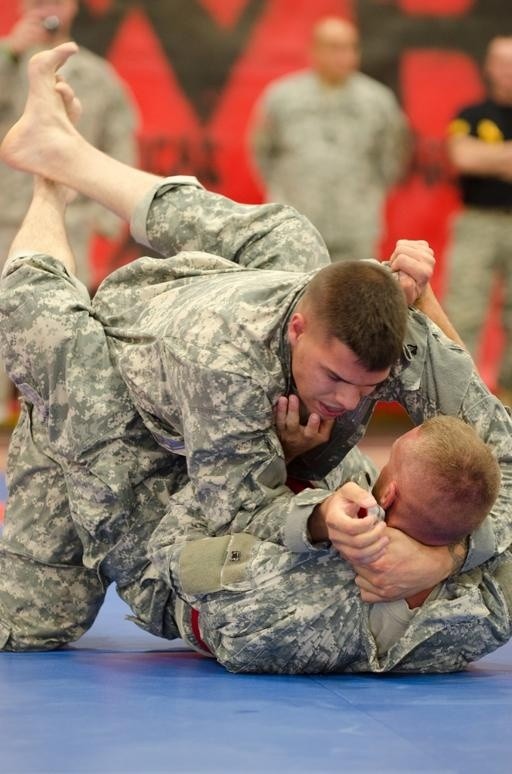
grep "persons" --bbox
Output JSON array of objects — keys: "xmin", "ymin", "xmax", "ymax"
[
  {"xmin": 1, "ymin": 39, "xmax": 512, "ymax": 678},
  {"xmin": 3, "ymin": 249, "xmax": 512, "ymax": 656},
  {"xmin": 434, "ymin": 33, "xmax": 512, "ymax": 426},
  {"xmin": 1, "ymin": 0, "xmax": 141, "ymax": 434},
  {"xmin": 244, "ymin": 15, "xmax": 418, "ymax": 268}
]
